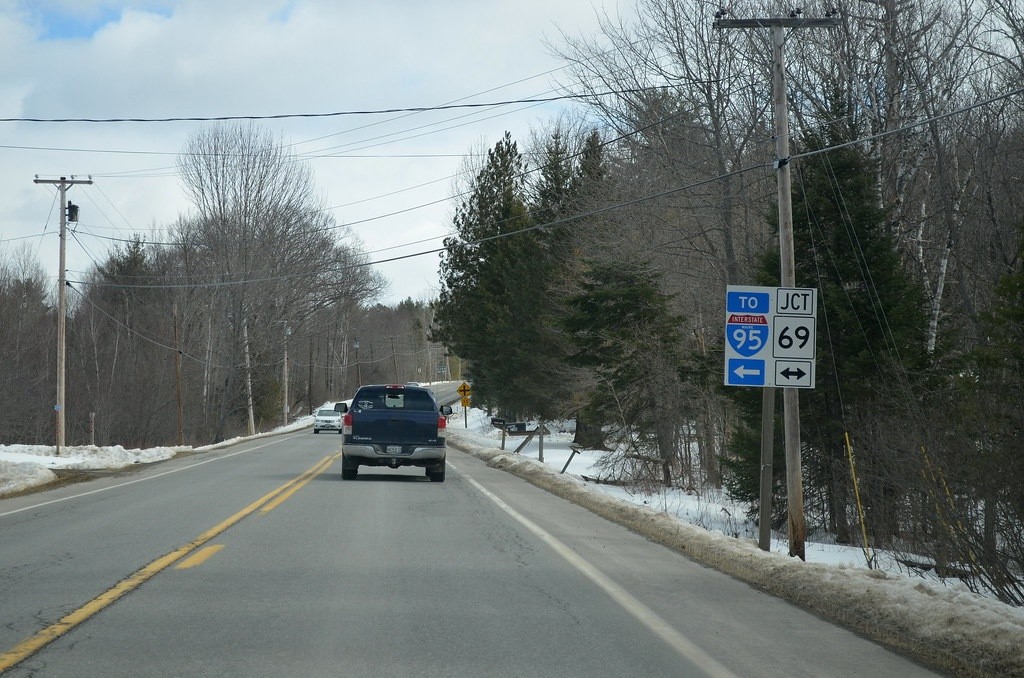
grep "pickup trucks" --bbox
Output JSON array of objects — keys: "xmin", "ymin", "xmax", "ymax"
[{"xmin": 334, "ymin": 384, "xmax": 453, "ymax": 482}]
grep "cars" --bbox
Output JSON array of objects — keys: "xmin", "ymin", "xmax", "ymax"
[{"xmin": 313, "ymin": 407, "xmax": 346, "ymax": 434}]
